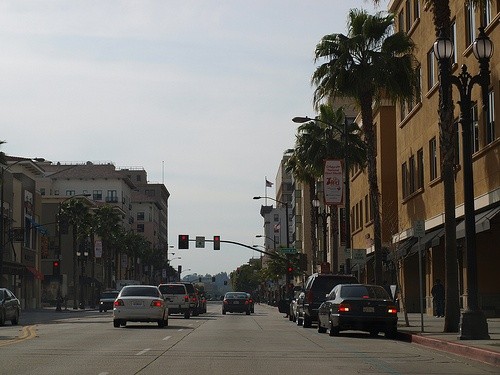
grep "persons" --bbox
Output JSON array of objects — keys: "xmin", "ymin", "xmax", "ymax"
[{"xmin": 430, "ymin": 279, "xmax": 444, "ymax": 317}]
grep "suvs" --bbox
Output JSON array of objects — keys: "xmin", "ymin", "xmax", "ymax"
[
  {"xmin": 98, "ymin": 291, "xmax": 120, "ymax": 312},
  {"xmin": 292, "ymin": 272, "xmax": 361, "ymax": 328},
  {"xmin": 172, "ymin": 282, "xmax": 207, "ymax": 316},
  {"xmin": 157, "ymin": 283, "xmax": 192, "ymax": 319}
]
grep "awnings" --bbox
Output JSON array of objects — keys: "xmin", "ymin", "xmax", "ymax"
[
  {"xmin": 351, "ymin": 255, "xmax": 374, "ymax": 271},
  {"xmin": 26, "ymin": 267, "xmax": 43, "ymax": 279},
  {"xmin": 456, "ymin": 207, "xmax": 500, "ymax": 239},
  {"xmin": 387, "ymin": 227, "xmax": 445, "ymax": 260}
]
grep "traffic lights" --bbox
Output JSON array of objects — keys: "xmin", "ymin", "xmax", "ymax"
[
  {"xmin": 53, "ymin": 261, "xmax": 60, "ymax": 274},
  {"xmin": 214, "ymin": 235, "xmax": 220, "ymax": 250},
  {"xmin": 288, "ymin": 267, "xmax": 294, "ymax": 280},
  {"xmin": 178, "ymin": 235, "xmax": 189, "ymax": 249}
]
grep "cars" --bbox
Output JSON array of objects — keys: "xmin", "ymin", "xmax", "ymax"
[
  {"xmin": 0, "ymin": 288, "xmax": 21, "ymax": 325},
  {"xmin": 220, "ymin": 292, "xmax": 252, "ymax": 315},
  {"xmin": 246, "ymin": 294, "xmax": 255, "ymax": 313},
  {"xmin": 277, "ymin": 294, "xmax": 295, "ymax": 321},
  {"xmin": 112, "ymin": 284, "xmax": 171, "ymax": 329},
  {"xmin": 316, "ymin": 283, "xmax": 398, "ymax": 340}
]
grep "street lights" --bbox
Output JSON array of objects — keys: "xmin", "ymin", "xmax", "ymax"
[
  {"xmin": 311, "ymin": 195, "xmax": 332, "ymax": 272},
  {"xmin": 253, "ymin": 196, "xmax": 291, "ymax": 267},
  {"xmin": 256, "ymin": 233, "xmax": 277, "ymax": 266},
  {"xmin": 432, "ymin": 25, "xmax": 495, "ymax": 340},
  {"xmin": 76, "ymin": 248, "xmax": 89, "ymax": 309},
  {"xmin": 55, "ymin": 193, "xmax": 92, "ymax": 311},
  {"xmin": 292, "ymin": 117, "xmax": 383, "ymax": 283}
]
